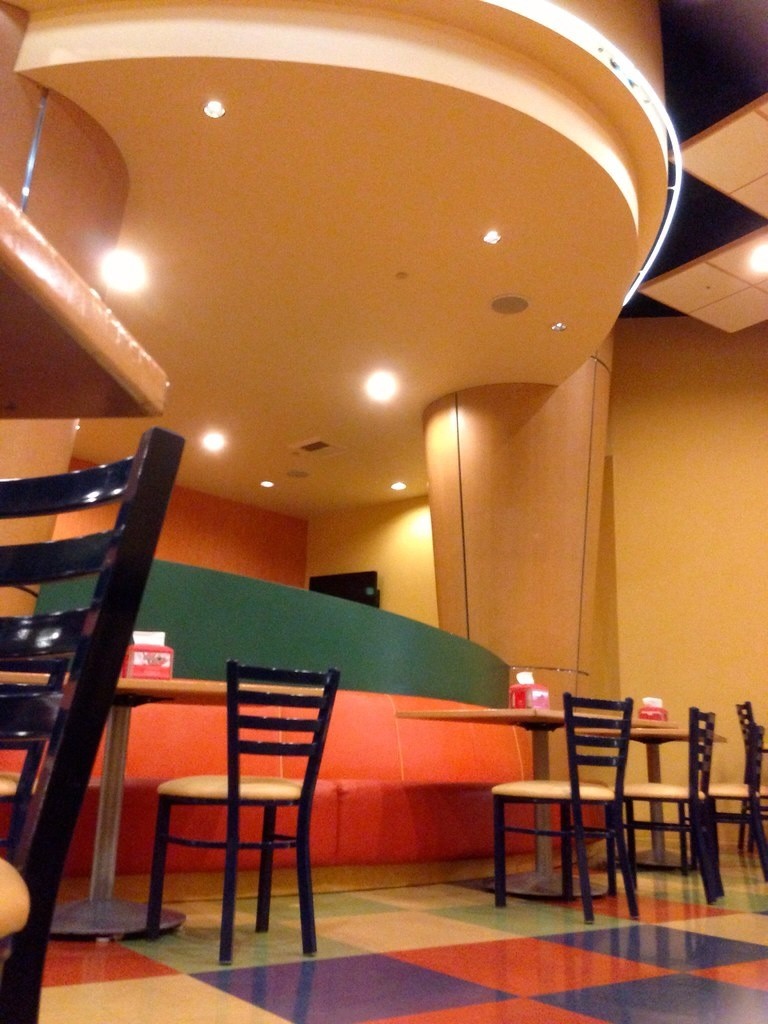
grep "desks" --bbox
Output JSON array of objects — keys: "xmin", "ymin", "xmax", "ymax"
[
  {"xmin": 393, "ymin": 707, "xmax": 679, "ymax": 899},
  {"xmin": 588, "ymin": 729, "xmax": 728, "ymax": 873},
  {"xmin": 0, "ymin": 671, "xmax": 323, "ymax": 942}
]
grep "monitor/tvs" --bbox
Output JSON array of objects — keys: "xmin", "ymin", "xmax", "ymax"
[{"xmin": 308, "ymin": 570, "xmax": 378, "ymax": 610}]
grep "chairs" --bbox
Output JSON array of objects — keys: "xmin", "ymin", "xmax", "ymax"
[
  {"xmin": 145, "ymin": 662, "xmax": 342, "ymax": 965},
  {"xmin": 491, "ymin": 696, "xmax": 642, "ymax": 924},
  {"xmin": 605, "ymin": 709, "xmax": 724, "ymax": 906},
  {"xmin": 1, "ymin": 424, "xmax": 183, "ymax": 1023},
  {"xmin": 695, "ymin": 703, "xmax": 768, "ymax": 882}
]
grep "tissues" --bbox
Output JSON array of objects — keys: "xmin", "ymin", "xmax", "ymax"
[
  {"xmin": 508, "ymin": 671, "xmax": 550, "ymax": 709},
  {"xmin": 639, "ymin": 697, "xmax": 668, "ymax": 721},
  {"xmin": 122, "ymin": 631, "xmax": 174, "ymax": 680}
]
